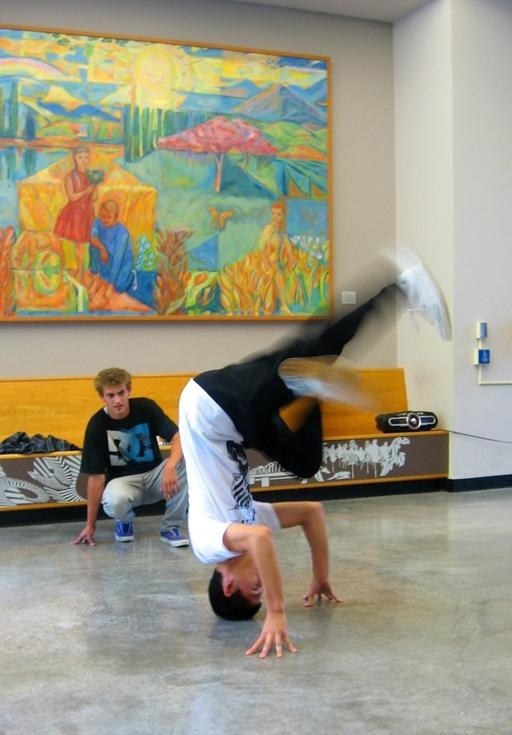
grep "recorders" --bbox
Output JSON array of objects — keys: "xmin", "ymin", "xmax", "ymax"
[{"xmin": 376, "ymin": 411, "xmax": 437, "ymax": 432}]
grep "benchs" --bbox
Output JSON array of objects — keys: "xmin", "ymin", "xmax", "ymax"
[{"xmin": 0, "ymin": 368, "xmax": 449, "ymax": 512}]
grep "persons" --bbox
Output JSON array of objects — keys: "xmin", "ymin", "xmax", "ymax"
[
  {"xmin": 177, "ymin": 261, "xmax": 454, "ymax": 662},
  {"xmin": 69, "ymin": 367, "xmax": 190, "ymax": 550}
]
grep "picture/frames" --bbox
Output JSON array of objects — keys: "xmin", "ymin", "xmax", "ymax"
[{"xmin": 1, "ymin": 24, "xmax": 335, "ymax": 323}]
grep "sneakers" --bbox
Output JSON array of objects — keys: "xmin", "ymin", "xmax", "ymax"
[
  {"xmin": 278, "ymin": 355, "xmax": 377, "ymax": 412},
  {"xmin": 391, "ymin": 261, "xmax": 454, "ymax": 342},
  {"xmin": 114, "ymin": 519, "xmax": 135, "ymax": 542},
  {"xmin": 159, "ymin": 526, "xmax": 190, "ymax": 548}
]
